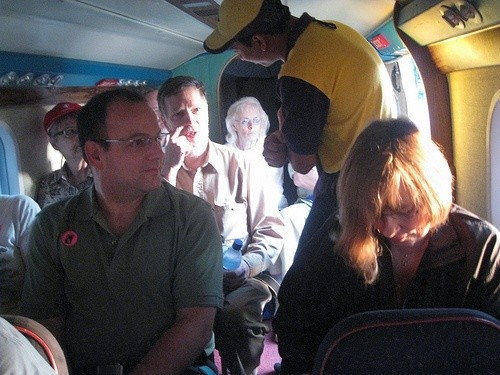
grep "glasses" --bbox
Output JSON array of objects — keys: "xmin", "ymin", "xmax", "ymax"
[
  {"xmin": 93, "ymin": 133, "xmax": 171, "ymax": 151},
  {"xmin": 234, "ymin": 117, "xmax": 261, "ymax": 125},
  {"xmin": 51, "ymin": 127, "xmax": 78, "ymax": 138},
  {"xmin": 379, "ymin": 210, "xmax": 415, "ymax": 218}
]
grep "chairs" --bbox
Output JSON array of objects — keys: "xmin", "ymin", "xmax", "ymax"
[{"xmin": 313, "ymin": 304, "xmax": 500, "ymax": 375}]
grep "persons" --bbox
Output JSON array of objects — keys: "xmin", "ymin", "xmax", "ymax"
[
  {"xmin": 21, "ymin": 89, "xmax": 224, "ymax": 375},
  {"xmin": 157, "ymin": 76, "xmax": 287, "ymax": 375},
  {"xmin": 273, "ymin": 117, "xmax": 500, "ymax": 375},
  {"xmin": 0, "ymin": 194, "xmax": 57, "ymax": 375},
  {"xmin": 223, "ymin": 96, "xmax": 319, "ymax": 281},
  {"xmin": 142, "ymin": 85, "xmax": 168, "ymax": 130},
  {"xmin": 203, "ymin": 0, "xmax": 398, "ymax": 371},
  {"xmin": 36, "ymin": 102, "xmax": 94, "ymax": 208}
]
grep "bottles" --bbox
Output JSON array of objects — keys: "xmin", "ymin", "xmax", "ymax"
[{"xmin": 223, "ymin": 238, "xmax": 244, "ymax": 271}]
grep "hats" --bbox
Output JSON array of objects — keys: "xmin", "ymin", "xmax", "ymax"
[
  {"xmin": 203, "ymin": 0, "xmax": 281, "ymax": 54},
  {"xmin": 44, "ymin": 103, "xmax": 82, "ymax": 135}
]
